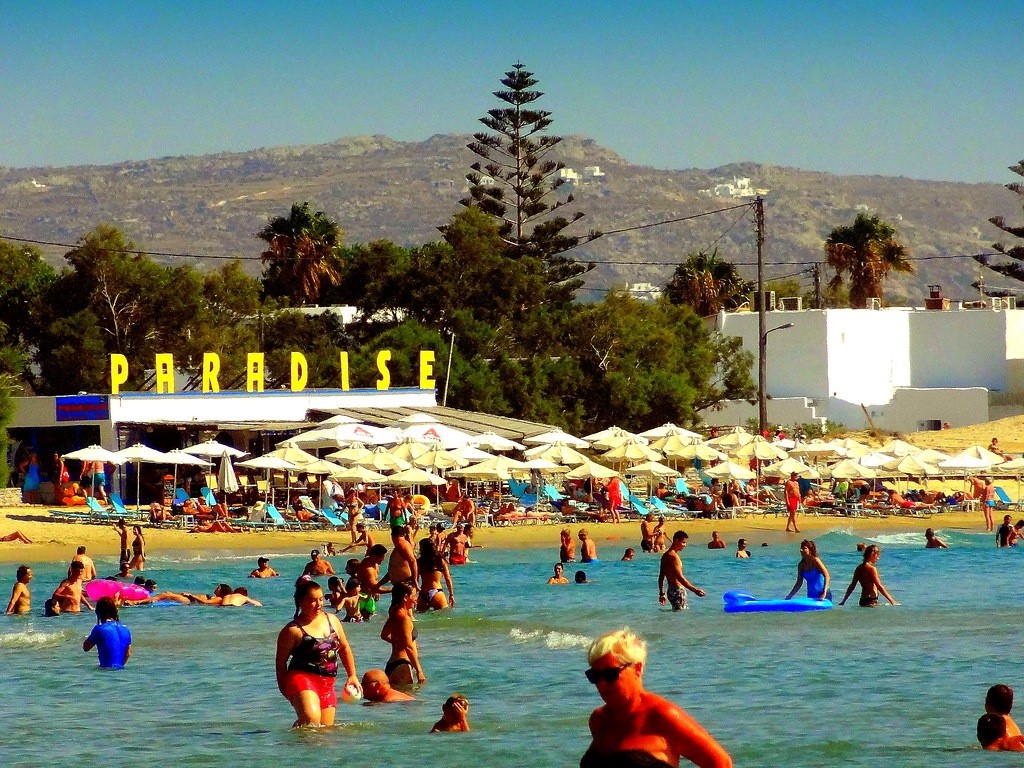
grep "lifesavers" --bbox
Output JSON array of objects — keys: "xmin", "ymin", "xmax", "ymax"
[
  {"xmin": 723, "ymin": 589, "xmax": 834, "ymax": 612},
  {"xmin": 85, "ymin": 580, "xmax": 149, "ymax": 604}
]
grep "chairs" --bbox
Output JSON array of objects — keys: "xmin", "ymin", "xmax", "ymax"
[{"xmin": 48, "ymin": 476, "xmax": 1024, "ymax": 529}]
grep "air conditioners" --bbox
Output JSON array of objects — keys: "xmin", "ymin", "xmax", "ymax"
[
  {"xmin": 750, "ymin": 291, "xmax": 775, "ymax": 311},
  {"xmin": 780, "ymin": 297, "xmax": 802, "ymax": 311},
  {"xmin": 866, "ymin": 298, "xmax": 881, "ymax": 309},
  {"xmin": 987, "ymin": 297, "xmax": 1016, "ymax": 310}
]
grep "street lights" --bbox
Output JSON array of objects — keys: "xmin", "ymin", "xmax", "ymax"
[{"xmin": 759, "ymin": 322, "xmax": 795, "ymax": 434}]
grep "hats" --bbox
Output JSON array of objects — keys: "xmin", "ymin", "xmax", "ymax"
[
  {"xmin": 257, "ymin": 557, "xmax": 270, "ymax": 565},
  {"xmin": 311, "ymin": 550, "xmax": 319, "ymax": 556}
]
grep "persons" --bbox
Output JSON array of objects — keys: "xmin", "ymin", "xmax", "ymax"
[
  {"xmin": 8, "ymin": 496, "xmax": 279, "ymax": 670},
  {"xmin": 20, "ymin": 451, "xmax": 107, "ymax": 505},
  {"xmin": 580, "ymin": 629, "xmax": 732, "ymax": 768},
  {"xmin": 788, "ymin": 436, "xmax": 1023, "ymax": 608},
  {"xmin": 547, "ymin": 426, "xmax": 799, "ymax": 607},
  {"xmin": 978, "ymin": 684, "xmax": 1024, "ymax": 751},
  {"xmin": 273, "ymin": 466, "xmax": 545, "ymax": 730}
]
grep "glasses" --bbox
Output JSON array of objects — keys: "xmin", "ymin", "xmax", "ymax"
[{"xmin": 586, "ymin": 662, "xmax": 633, "ymax": 684}]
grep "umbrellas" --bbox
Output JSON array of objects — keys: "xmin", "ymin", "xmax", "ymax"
[{"xmin": 58, "ymin": 412, "xmax": 1024, "ymax": 528}]
